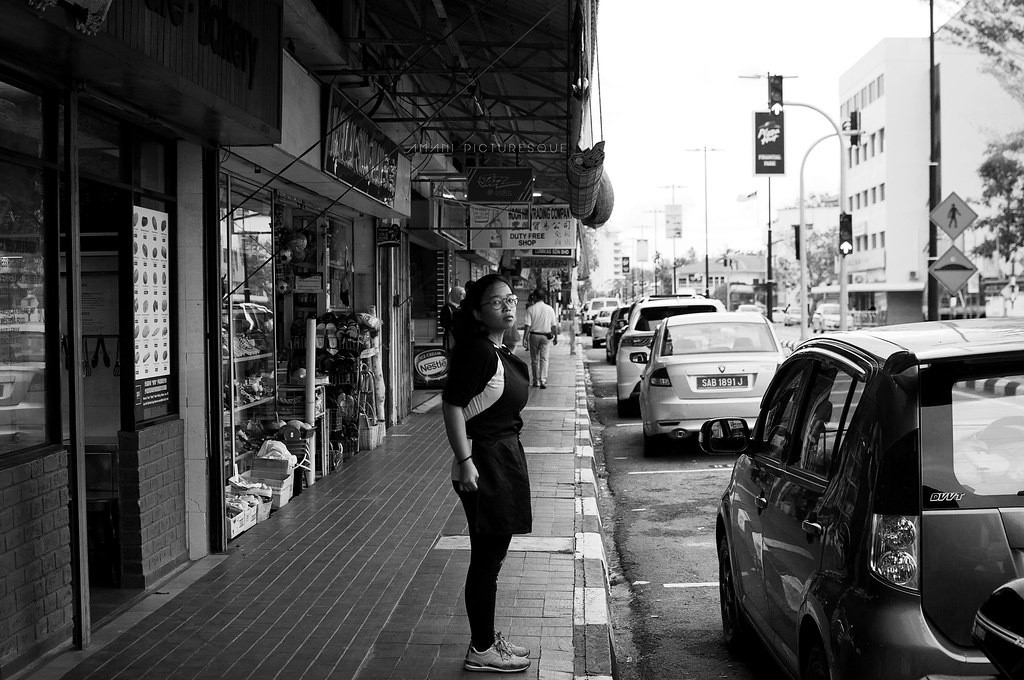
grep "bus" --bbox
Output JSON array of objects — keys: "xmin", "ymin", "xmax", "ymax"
[{"xmin": 728, "ymin": 285, "xmax": 757, "ymax": 312}]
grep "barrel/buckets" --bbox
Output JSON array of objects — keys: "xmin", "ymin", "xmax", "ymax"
[{"xmin": 282, "ymin": 439, "xmax": 312, "ymax": 496}]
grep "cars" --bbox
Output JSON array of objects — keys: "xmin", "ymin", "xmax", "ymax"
[
  {"xmin": 591, "ymin": 293, "xmax": 728, "ymax": 419},
  {"xmin": 737, "ymin": 304, "xmax": 762, "ymax": 314},
  {"xmin": 716, "ymin": 318, "xmax": 1024, "ymax": 680},
  {"xmin": 812, "ymin": 304, "xmax": 856, "ymax": 334},
  {"xmin": 628, "ymin": 313, "xmax": 789, "ymax": 452},
  {"xmin": 772, "ymin": 305, "xmax": 811, "ymax": 328}
]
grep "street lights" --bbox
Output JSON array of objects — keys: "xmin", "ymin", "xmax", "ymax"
[
  {"xmin": 647, "ymin": 183, "xmax": 682, "ymax": 294},
  {"xmin": 780, "ymin": 101, "xmax": 850, "ymax": 330},
  {"xmin": 798, "ymin": 130, "xmax": 868, "ymax": 339},
  {"xmin": 695, "ymin": 146, "xmax": 716, "ymax": 298}
]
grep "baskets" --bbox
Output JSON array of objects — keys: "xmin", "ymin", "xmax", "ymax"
[{"xmin": 358, "ymin": 398, "xmax": 384, "ymax": 451}]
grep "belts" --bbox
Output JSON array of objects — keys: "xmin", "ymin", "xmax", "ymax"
[{"xmin": 529, "ymin": 331, "xmax": 548, "ymax": 336}]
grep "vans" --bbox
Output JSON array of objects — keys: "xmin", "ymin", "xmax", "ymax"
[{"xmin": 585, "ymin": 299, "xmax": 626, "ymax": 335}]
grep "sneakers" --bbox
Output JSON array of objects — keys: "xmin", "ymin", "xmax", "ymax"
[
  {"xmin": 465, "ymin": 644, "xmax": 530, "ymax": 672},
  {"xmin": 494, "ymin": 631, "xmax": 530, "ymax": 658}
]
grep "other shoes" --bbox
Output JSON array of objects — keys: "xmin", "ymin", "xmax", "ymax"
[
  {"xmin": 287, "ymin": 310, "xmax": 378, "ymax": 456},
  {"xmin": 221, "ymin": 326, "xmax": 281, "ymax": 462},
  {"xmin": 540, "ymin": 382, "xmax": 546, "ymax": 389},
  {"xmin": 533, "ymin": 379, "xmax": 541, "ymax": 387}
]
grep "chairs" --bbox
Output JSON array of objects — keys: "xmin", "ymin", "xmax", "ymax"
[
  {"xmin": 673, "ymin": 338, "xmax": 696, "ymax": 354},
  {"xmin": 731, "ymin": 337, "xmax": 755, "ymax": 351}
]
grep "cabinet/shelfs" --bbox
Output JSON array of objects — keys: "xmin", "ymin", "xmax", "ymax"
[{"xmin": 222, "ymin": 266, "xmax": 373, "ymax": 488}]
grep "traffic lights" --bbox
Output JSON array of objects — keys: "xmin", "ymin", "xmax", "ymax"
[{"xmin": 839, "ymin": 213, "xmax": 854, "ymax": 255}]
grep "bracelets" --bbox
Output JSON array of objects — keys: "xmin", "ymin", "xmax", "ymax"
[{"xmin": 457, "ymin": 455, "xmax": 471, "ymax": 465}]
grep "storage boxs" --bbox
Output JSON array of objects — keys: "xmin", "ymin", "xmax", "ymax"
[
  {"xmin": 358, "ymin": 405, "xmax": 378, "ymax": 450},
  {"xmin": 225, "ymin": 457, "xmax": 294, "ymax": 539},
  {"xmin": 361, "ymin": 401, "xmax": 385, "ymax": 447}
]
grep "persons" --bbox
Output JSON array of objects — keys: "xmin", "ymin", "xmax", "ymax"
[
  {"xmin": 868, "ymin": 304, "xmax": 876, "ymax": 310},
  {"xmin": 442, "ymin": 274, "xmax": 534, "ymax": 672},
  {"xmin": 441, "ymin": 280, "xmax": 521, "ymax": 354},
  {"xmin": 523, "ymin": 287, "xmax": 558, "ymax": 389}
]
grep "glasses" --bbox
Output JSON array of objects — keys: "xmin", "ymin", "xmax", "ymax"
[{"xmin": 477, "ymin": 294, "xmax": 520, "ymax": 310}]
